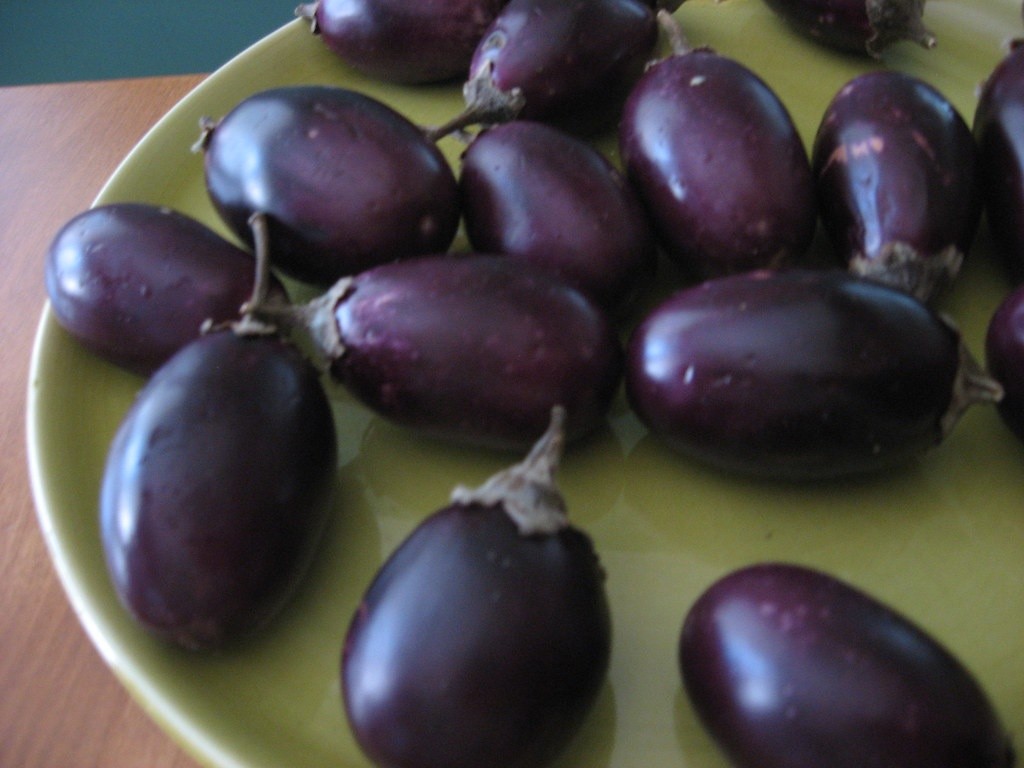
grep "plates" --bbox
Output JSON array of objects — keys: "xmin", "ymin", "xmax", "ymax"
[{"xmin": 27, "ymin": 0, "xmax": 1024, "ymax": 768}]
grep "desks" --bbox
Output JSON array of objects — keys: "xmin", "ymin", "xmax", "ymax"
[{"xmin": 0, "ymin": 73, "xmax": 212, "ymax": 768}]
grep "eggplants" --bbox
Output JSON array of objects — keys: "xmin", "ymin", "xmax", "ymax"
[{"xmin": 40, "ymin": 0, "xmax": 1024, "ymax": 767}]
